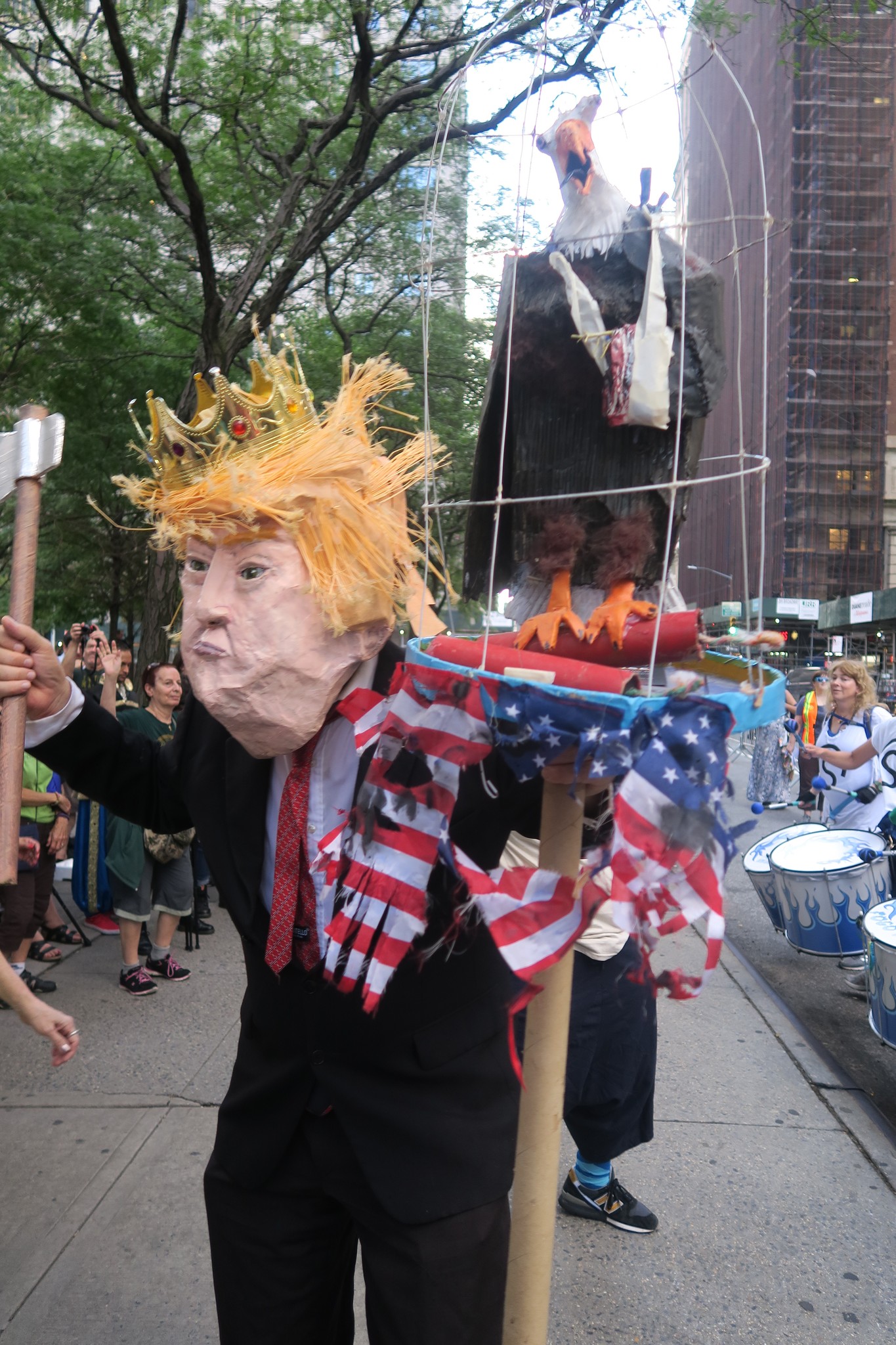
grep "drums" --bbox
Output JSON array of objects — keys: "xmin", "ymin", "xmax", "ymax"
[
  {"xmin": 767, "ymin": 828, "xmax": 894, "ymax": 958},
  {"xmin": 741, "ymin": 821, "xmax": 830, "ymax": 933},
  {"xmin": 862, "ymin": 898, "xmax": 896, "ymax": 1050}
]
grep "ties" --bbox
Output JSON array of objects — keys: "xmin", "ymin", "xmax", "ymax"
[{"xmin": 264, "ymin": 703, "xmax": 347, "ymax": 975}]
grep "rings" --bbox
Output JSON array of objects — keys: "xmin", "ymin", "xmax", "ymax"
[{"xmin": 67, "ymin": 1029, "xmax": 79, "ymax": 1038}]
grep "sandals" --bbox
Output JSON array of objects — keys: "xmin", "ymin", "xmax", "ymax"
[
  {"xmin": 27, "ymin": 940, "xmax": 62, "ymax": 962},
  {"xmin": 41, "ymin": 925, "xmax": 81, "ymax": 944}
]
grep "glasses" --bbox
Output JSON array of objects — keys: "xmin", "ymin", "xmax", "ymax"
[{"xmin": 815, "ymin": 677, "xmax": 830, "ymax": 682}]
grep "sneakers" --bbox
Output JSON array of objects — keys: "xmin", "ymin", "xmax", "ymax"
[
  {"xmin": 118, "ymin": 965, "xmax": 158, "ymax": 996},
  {"xmin": 558, "ymin": 1166, "xmax": 659, "ymax": 1234},
  {"xmin": 144, "ymin": 950, "xmax": 191, "ymax": 981}
]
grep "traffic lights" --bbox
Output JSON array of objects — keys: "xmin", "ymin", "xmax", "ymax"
[{"xmin": 729, "ymin": 617, "xmax": 738, "ymax": 636}]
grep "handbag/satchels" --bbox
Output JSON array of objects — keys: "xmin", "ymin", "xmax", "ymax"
[{"xmin": 144, "ymin": 828, "xmax": 197, "ymax": 865}]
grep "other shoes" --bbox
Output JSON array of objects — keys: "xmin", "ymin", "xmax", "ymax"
[
  {"xmin": 839, "ymin": 954, "xmax": 868, "ymax": 970},
  {"xmin": 844, "ymin": 971, "xmax": 870, "ymax": 992},
  {"xmin": 83, "ymin": 914, "xmax": 120, "ymax": 934},
  {"xmin": 9, "ymin": 970, "xmax": 55, "ymax": 993}
]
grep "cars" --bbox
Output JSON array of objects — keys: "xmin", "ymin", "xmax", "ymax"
[{"xmin": 782, "ymin": 666, "xmax": 824, "ymax": 699}]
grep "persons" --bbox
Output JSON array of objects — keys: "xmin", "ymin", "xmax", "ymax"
[
  {"xmin": 0, "ymin": 315, "xmax": 607, "ymax": 1345},
  {"xmin": 0, "ymin": 490, "xmax": 896, "ymax": 1235}
]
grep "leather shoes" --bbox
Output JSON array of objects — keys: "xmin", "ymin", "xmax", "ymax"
[
  {"xmin": 178, "ymin": 915, "xmax": 215, "ymax": 935},
  {"xmin": 137, "ymin": 923, "xmax": 153, "ymax": 955},
  {"xmin": 195, "ymin": 886, "xmax": 211, "ymax": 918}
]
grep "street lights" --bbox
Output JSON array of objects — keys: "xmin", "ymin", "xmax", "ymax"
[{"xmin": 686, "ymin": 565, "xmax": 734, "ymax": 655}]
